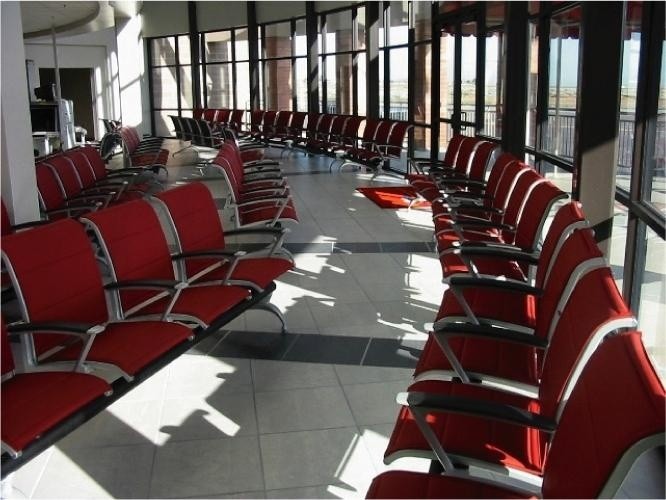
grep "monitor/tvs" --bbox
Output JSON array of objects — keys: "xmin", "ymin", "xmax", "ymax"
[{"xmin": 30, "ymin": 102, "xmax": 62, "ymax": 137}]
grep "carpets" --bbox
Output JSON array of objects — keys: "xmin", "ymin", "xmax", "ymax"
[{"xmin": 356, "ymin": 187, "xmax": 432, "ymax": 209}]
